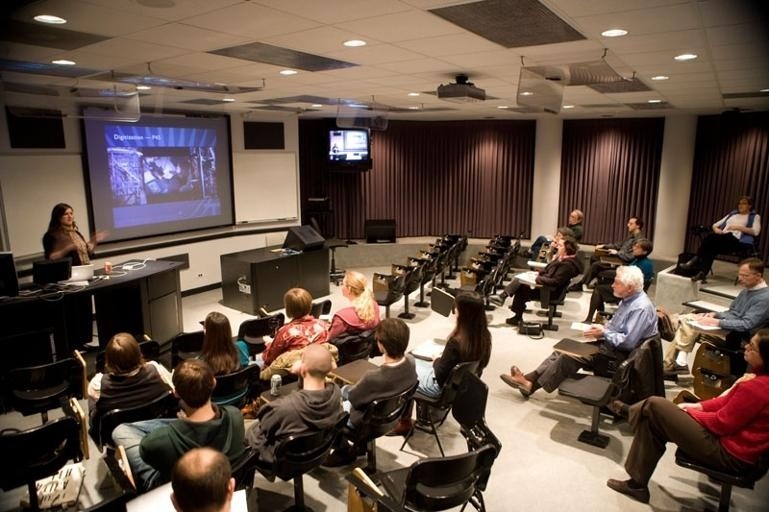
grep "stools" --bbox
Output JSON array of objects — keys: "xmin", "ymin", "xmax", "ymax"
[{"xmin": 329, "ymin": 240, "xmax": 347, "ymax": 287}]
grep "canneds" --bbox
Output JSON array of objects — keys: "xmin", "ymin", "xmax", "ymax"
[
  {"xmin": 271, "ymin": 374, "xmax": 282, "ymax": 396},
  {"xmin": 105, "ymin": 261, "xmax": 112, "ymax": 275}
]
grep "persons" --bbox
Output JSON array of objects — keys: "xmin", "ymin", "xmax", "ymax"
[
  {"xmin": 86, "ymin": 332, "xmax": 175, "ymax": 438},
  {"xmin": 110, "ymin": 356, "xmax": 246, "ymax": 492},
  {"xmin": 42, "ymin": 203, "xmax": 107, "ymax": 351},
  {"xmin": 499, "ymin": 265, "xmax": 659, "ymax": 399},
  {"xmin": 323, "ymin": 317, "xmax": 419, "ymax": 467},
  {"xmin": 661, "ymin": 256, "xmax": 769, "ymax": 372},
  {"xmin": 175, "ymin": 311, "xmax": 249, "ymax": 376},
  {"xmin": 489, "ymin": 209, "xmax": 655, "ymax": 327},
  {"xmin": 245, "ymin": 345, "xmax": 343, "ymax": 483},
  {"xmin": 331, "ymin": 270, "xmax": 380, "ymax": 342},
  {"xmin": 414, "ymin": 289, "xmax": 491, "ymax": 398},
  {"xmin": 607, "ymin": 326, "xmax": 768, "ymax": 504},
  {"xmin": 679, "ymin": 196, "xmax": 762, "ymax": 281},
  {"xmin": 254, "ymin": 287, "xmax": 333, "ymax": 373},
  {"xmin": 169, "ymin": 445, "xmax": 237, "ymax": 512}
]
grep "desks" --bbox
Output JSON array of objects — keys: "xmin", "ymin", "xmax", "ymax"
[
  {"xmin": 221, "ymin": 226, "xmax": 329, "ymax": 317},
  {"xmin": 1, "ymin": 260, "xmax": 184, "ymax": 361}
]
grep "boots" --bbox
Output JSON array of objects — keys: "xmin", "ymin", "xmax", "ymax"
[
  {"xmin": 691, "ymin": 265, "xmax": 708, "ymax": 281},
  {"xmin": 679, "ymin": 254, "xmax": 699, "ymax": 272}
]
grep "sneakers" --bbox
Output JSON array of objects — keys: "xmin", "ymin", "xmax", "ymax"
[
  {"xmin": 663, "ymin": 374, "xmax": 678, "ymax": 380},
  {"xmin": 488, "ymin": 294, "xmax": 505, "ymax": 307},
  {"xmin": 663, "ymin": 360, "xmax": 691, "ymax": 375},
  {"xmin": 385, "ymin": 417, "xmax": 415, "ymax": 436},
  {"xmin": 568, "ymin": 282, "xmax": 583, "ymax": 292},
  {"xmin": 506, "ymin": 316, "xmax": 524, "ymax": 326}
]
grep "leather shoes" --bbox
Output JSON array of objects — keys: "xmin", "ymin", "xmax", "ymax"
[
  {"xmin": 499, "ymin": 364, "xmax": 534, "ymax": 400},
  {"xmin": 611, "ymin": 399, "xmax": 631, "ymax": 418},
  {"xmin": 606, "ymin": 478, "xmax": 650, "ymax": 504}
]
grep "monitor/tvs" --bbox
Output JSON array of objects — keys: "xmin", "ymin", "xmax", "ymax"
[
  {"xmin": 33, "ymin": 258, "xmax": 71, "ymax": 293},
  {"xmin": 281, "ymin": 226, "xmax": 325, "ymax": 251},
  {"xmin": 327, "ymin": 127, "xmax": 370, "ymax": 169}
]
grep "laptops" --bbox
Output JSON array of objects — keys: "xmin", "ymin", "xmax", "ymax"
[{"xmin": 68, "ymin": 265, "xmax": 94, "ymax": 282}]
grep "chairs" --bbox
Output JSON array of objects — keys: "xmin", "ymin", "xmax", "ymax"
[
  {"xmin": 373, "ymin": 236, "xmax": 467, "ymax": 320},
  {"xmin": 1, "ymin": 300, "xmax": 496, "ymax": 512},
  {"xmin": 645, "ymin": 273, "xmax": 653, "ymax": 293},
  {"xmin": 524, "ymin": 241, "xmax": 564, "ymax": 331},
  {"xmin": 595, "ymin": 247, "xmax": 626, "ymax": 266},
  {"xmin": 462, "ymin": 235, "xmax": 521, "ymax": 306},
  {"xmin": 558, "ymin": 306, "xmax": 754, "ymax": 512}
]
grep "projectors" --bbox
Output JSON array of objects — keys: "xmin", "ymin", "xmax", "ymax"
[{"xmin": 438, "ymin": 84, "xmax": 485, "ymax": 104}]
